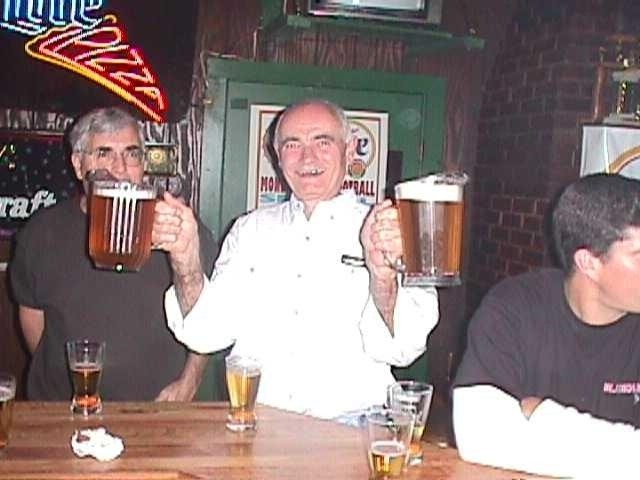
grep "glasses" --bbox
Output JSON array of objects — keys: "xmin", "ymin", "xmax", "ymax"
[{"xmin": 77, "ymin": 146, "xmax": 146, "ymax": 166}]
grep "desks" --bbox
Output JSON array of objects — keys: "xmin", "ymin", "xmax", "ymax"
[{"xmin": 0, "ymin": 401, "xmax": 547, "ymax": 480}]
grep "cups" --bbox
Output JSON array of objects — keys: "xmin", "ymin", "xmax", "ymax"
[
  {"xmin": 365, "ymin": 381, "xmax": 434, "ymax": 473},
  {"xmin": 384, "ymin": 169, "xmax": 469, "ymax": 288},
  {"xmin": 87, "ymin": 179, "xmax": 167, "ymax": 273},
  {"xmin": 67, "ymin": 338, "xmax": 106, "ymax": 416},
  {"xmin": 0, "ymin": 370, "xmax": 17, "ymax": 453},
  {"xmin": 224, "ymin": 353, "xmax": 261, "ymax": 431}
]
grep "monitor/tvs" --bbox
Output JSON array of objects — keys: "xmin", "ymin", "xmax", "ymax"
[{"xmin": 306, "ymin": 0, "xmax": 443, "ymax": 27}]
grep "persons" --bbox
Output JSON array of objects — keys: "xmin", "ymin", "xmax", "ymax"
[
  {"xmin": 150, "ymin": 100, "xmax": 440, "ymax": 421},
  {"xmin": 8, "ymin": 105, "xmax": 219, "ymax": 401},
  {"xmin": 449, "ymin": 172, "xmax": 640, "ymax": 479}
]
